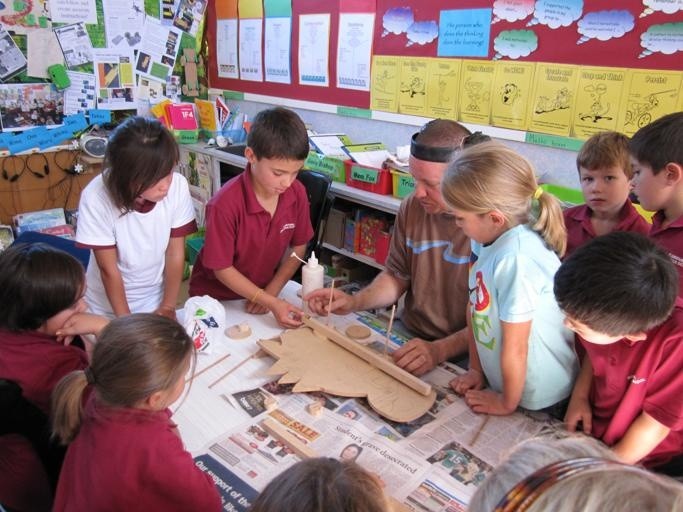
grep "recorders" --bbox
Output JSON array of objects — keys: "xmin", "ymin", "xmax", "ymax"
[{"xmin": 82, "ymin": 135, "xmax": 109, "ymax": 157}]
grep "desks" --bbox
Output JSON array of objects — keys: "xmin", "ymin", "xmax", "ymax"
[{"xmin": 72, "ymin": 275, "xmax": 681, "ymax": 512}]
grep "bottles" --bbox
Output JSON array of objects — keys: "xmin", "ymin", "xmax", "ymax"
[{"xmin": 300, "ymin": 250, "xmax": 325, "ymax": 318}]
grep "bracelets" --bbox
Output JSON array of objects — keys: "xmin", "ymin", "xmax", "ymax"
[
  {"xmin": 160, "ymin": 302, "xmax": 176, "ymax": 312},
  {"xmin": 251, "ymin": 288, "xmax": 265, "ymax": 302}
]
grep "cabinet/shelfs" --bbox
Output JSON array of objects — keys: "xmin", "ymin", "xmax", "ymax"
[{"xmin": 176, "ymin": 143, "xmax": 404, "ymax": 316}]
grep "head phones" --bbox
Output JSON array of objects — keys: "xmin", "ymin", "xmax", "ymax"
[
  {"xmin": 54, "ymin": 148, "xmax": 81, "ymax": 174},
  {"xmin": 1, "ymin": 154, "xmax": 25, "ymax": 181},
  {"xmin": 25, "ymin": 152, "xmax": 50, "ymax": 178}
]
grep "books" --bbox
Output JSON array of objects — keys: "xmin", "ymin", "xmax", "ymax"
[{"xmin": 150, "ymin": 95, "xmax": 411, "ymax": 174}]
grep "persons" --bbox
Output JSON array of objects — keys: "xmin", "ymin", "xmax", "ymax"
[
  {"xmin": 561, "ymin": 130, "xmax": 651, "ymax": 260},
  {"xmin": 440, "ymin": 140, "xmax": 579, "ymax": 421},
  {"xmin": 49, "ymin": 312, "xmax": 222, "ymax": 512},
  {"xmin": 304, "ymin": 118, "xmax": 492, "ymax": 376},
  {"xmin": 627, "ymin": 111, "xmax": 682, "ymax": 299},
  {"xmin": 552, "ymin": 231, "xmax": 683, "ymax": 469},
  {"xmin": 2, "ymin": 98, "xmax": 63, "ymax": 125},
  {"xmin": 187, "ymin": 106, "xmax": 315, "ymax": 329},
  {"xmin": 264, "ymin": 377, "xmax": 494, "ymax": 487},
  {"xmin": 0, "ymin": 241, "xmax": 113, "ymax": 511},
  {"xmin": 74, "ymin": 116, "xmax": 198, "ymax": 345},
  {"xmin": 248, "ymin": 458, "xmax": 416, "ymax": 512},
  {"xmin": 468, "ymin": 431, "xmax": 683, "ymax": 511}
]
